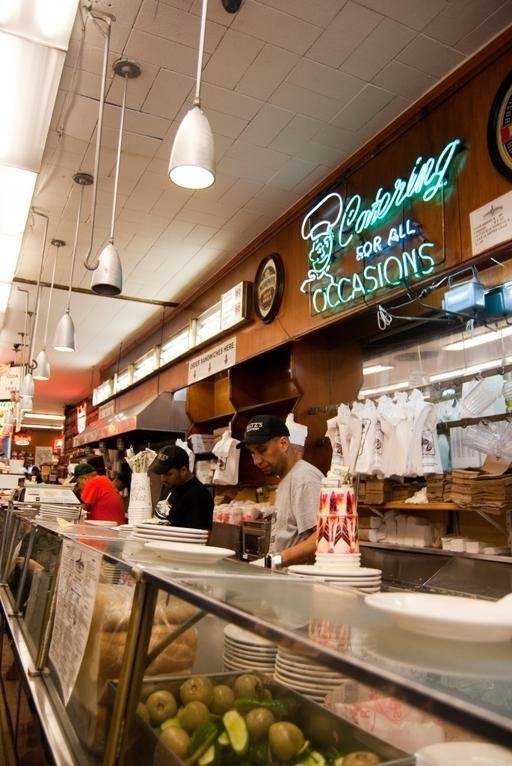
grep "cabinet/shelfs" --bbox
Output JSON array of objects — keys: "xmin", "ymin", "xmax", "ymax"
[{"xmin": 184, "ymin": 340, "xmax": 331, "ymax": 489}]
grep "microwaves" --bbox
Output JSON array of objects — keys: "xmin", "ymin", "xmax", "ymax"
[{"xmin": 241, "ymin": 519, "xmax": 271, "ymax": 562}]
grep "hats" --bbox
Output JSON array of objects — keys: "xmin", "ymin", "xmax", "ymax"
[
  {"xmin": 69, "ymin": 464, "xmax": 95, "ymax": 483},
  {"xmin": 148, "ymin": 446, "xmax": 190, "ymax": 476},
  {"xmin": 235, "ymin": 415, "xmax": 291, "ymax": 448}
]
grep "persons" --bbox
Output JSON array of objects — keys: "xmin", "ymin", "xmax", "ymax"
[
  {"xmin": 31, "ymin": 465, "xmax": 42, "ymax": 483},
  {"xmin": 149, "ymin": 445, "xmax": 214, "ymax": 545},
  {"xmin": 4, "ymin": 519, "xmax": 63, "ymax": 681},
  {"xmin": 67, "ymin": 464, "xmax": 125, "ymax": 528},
  {"xmin": 234, "ymin": 413, "xmax": 327, "ymax": 570}
]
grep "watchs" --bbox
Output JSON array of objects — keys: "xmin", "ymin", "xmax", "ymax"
[{"xmin": 272, "ymin": 551, "xmax": 284, "ymax": 570}]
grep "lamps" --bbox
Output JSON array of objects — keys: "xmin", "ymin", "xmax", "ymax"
[
  {"xmin": 91, "ymin": 58, "xmax": 142, "ymax": 298},
  {"xmin": 6, "ymin": 171, "xmax": 94, "ymax": 433},
  {"xmin": 166, "ymin": 0, "xmax": 216, "ymax": 189},
  {"xmin": 429, "ymin": 354, "xmax": 512, "ymax": 384},
  {"xmin": 359, "ymin": 381, "xmax": 408, "ymax": 395},
  {"xmin": 362, "ymin": 362, "xmax": 395, "ymax": 376},
  {"xmin": 441, "ymin": 324, "xmax": 512, "ymax": 350}
]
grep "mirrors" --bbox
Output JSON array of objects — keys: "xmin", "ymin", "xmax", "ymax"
[{"xmin": 358, "ymin": 317, "xmax": 512, "ymax": 485}]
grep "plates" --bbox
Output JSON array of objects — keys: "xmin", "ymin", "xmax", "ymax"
[
  {"xmin": 286, "ymin": 563, "xmax": 383, "ymax": 596},
  {"xmin": 120, "ymin": 539, "xmax": 158, "ymax": 566},
  {"xmin": 84, "ymin": 519, "xmax": 117, "ymax": 527},
  {"xmin": 411, "ymin": 740, "xmax": 511, "ymax": 766},
  {"xmin": 361, "ymin": 626, "xmax": 511, "ymax": 681},
  {"xmin": 143, "ymin": 541, "xmax": 237, "ymax": 565},
  {"xmin": 219, "ymin": 621, "xmax": 356, "ymax": 708},
  {"xmin": 363, "ymin": 589, "xmax": 511, "ymax": 644},
  {"xmin": 116, "ymin": 522, "xmax": 209, "ymax": 547},
  {"xmin": 37, "ymin": 503, "xmax": 81, "ymax": 523},
  {"xmin": 18, "ymin": 507, "xmax": 37, "ymax": 511}
]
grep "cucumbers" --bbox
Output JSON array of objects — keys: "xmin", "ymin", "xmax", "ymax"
[{"xmin": 183, "ymin": 696, "xmax": 329, "ymax": 766}]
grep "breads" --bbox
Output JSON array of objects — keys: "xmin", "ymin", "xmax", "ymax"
[{"xmin": 68, "ymin": 583, "xmax": 199, "ymax": 757}]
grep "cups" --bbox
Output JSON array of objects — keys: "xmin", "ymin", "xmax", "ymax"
[
  {"xmin": 124, "ymin": 472, "xmax": 154, "ymax": 526},
  {"xmin": 311, "ymin": 486, "xmax": 362, "ymax": 572}
]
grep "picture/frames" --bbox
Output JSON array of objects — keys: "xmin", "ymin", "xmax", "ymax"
[{"xmin": 253, "ymin": 252, "xmax": 284, "ymax": 323}]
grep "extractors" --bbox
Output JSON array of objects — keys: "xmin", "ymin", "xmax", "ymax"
[{"xmin": 73, "ymin": 391, "xmax": 191, "ymax": 449}]
